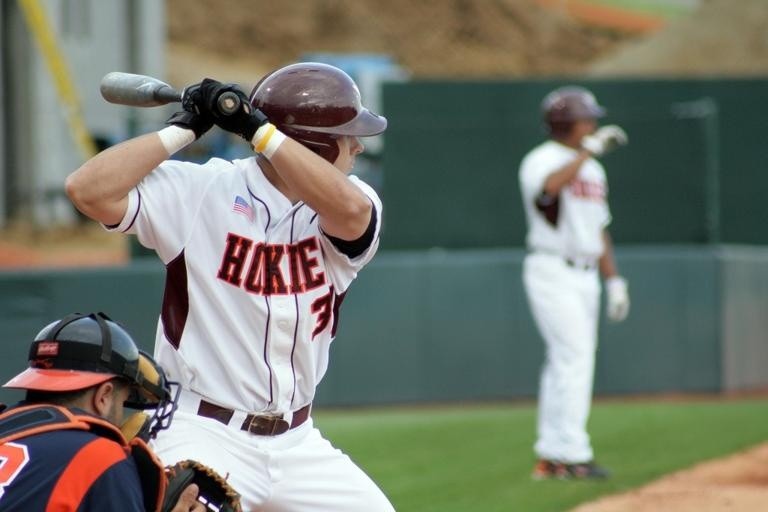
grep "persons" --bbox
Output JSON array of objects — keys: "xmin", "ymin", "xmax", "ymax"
[
  {"xmin": 63, "ymin": 60, "xmax": 396, "ymax": 512},
  {"xmin": 0, "ymin": 313, "xmax": 208, "ymax": 511},
  {"xmin": 516, "ymin": 84, "xmax": 631, "ymax": 483}
]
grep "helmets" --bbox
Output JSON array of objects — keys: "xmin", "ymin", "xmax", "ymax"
[
  {"xmin": 543, "ymin": 88, "xmax": 605, "ymax": 122},
  {"xmin": 247, "ymin": 62, "xmax": 388, "ymax": 165},
  {"xmin": 1, "ymin": 316, "xmax": 182, "ymax": 440}
]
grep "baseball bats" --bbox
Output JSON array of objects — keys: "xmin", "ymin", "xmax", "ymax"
[{"xmin": 99, "ymin": 71, "xmax": 240, "ymax": 116}]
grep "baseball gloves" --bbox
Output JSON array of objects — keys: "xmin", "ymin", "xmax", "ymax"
[{"xmin": 162, "ymin": 460, "xmax": 244, "ymax": 512}]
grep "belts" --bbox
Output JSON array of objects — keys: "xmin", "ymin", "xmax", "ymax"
[{"xmin": 197, "ymin": 399, "xmax": 310, "ymax": 437}]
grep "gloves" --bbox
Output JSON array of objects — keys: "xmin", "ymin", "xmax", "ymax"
[
  {"xmin": 166, "ymin": 78, "xmax": 270, "ymax": 142},
  {"xmin": 582, "ymin": 125, "xmax": 628, "ymax": 158},
  {"xmin": 604, "ymin": 280, "xmax": 629, "ymax": 323}
]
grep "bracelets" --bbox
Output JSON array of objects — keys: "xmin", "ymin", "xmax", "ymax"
[{"xmin": 250, "ymin": 122, "xmax": 288, "ymax": 161}]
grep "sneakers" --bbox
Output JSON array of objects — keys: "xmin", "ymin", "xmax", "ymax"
[{"xmin": 530, "ymin": 460, "xmax": 614, "ymax": 482}]
